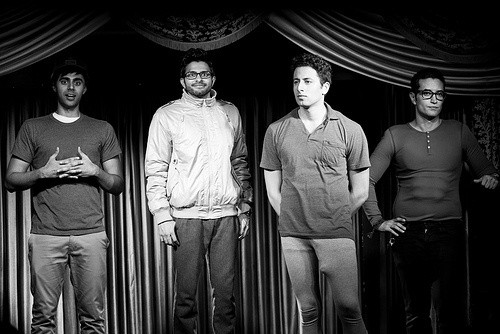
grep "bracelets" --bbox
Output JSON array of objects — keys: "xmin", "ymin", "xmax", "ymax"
[{"xmin": 242, "ymin": 209, "xmax": 252, "ymax": 220}]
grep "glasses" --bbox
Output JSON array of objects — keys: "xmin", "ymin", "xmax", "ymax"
[
  {"xmin": 183, "ymin": 71, "xmax": 212, "ymax": 80},
  {"xmin": 416, "ymin": 90, "xmax": 447, "ymax": 101}
]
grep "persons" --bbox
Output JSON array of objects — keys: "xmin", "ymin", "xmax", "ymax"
[
  {"xmin": 4, "ymin": 64, "xmax": 124, "ymax": 334},
  {"xmin": 259, "ymin": 54, "xmax": 372, "ymax": 334},
  {"xmin": 144, "ymin": 49, "xmax": 252, "ymax": 334},
  {"xmin": 361, "ymin": 68, "xmax": 500, "ymax": 334}
]
